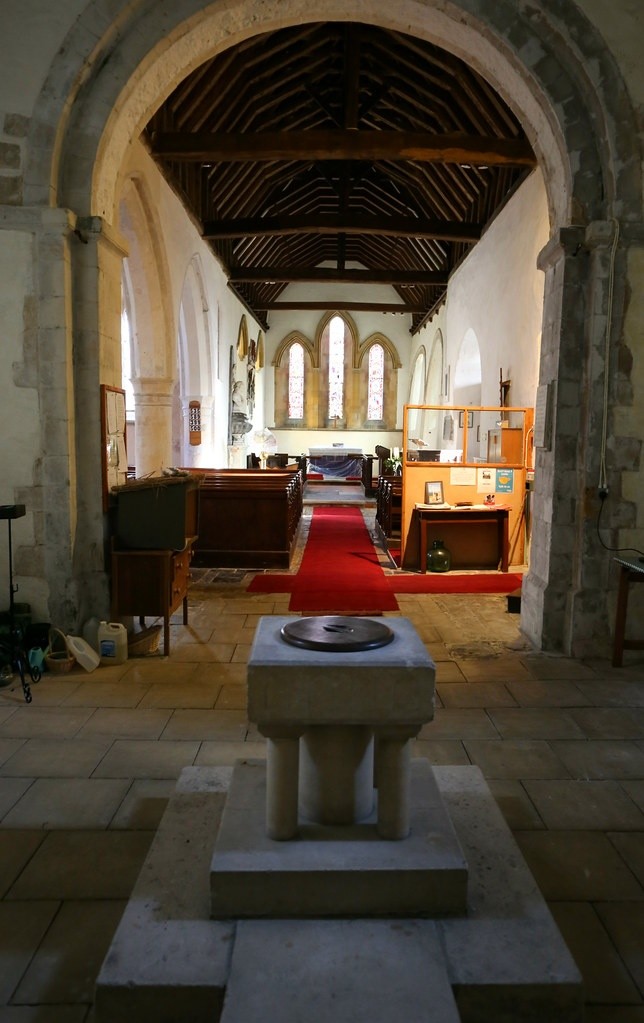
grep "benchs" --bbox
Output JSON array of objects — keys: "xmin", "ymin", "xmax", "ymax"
[
  {"xmin": 375, "ymin": 475, "xmax": 404, "ymax": 549},
  {"xmin": 176, "ymin": 467, "xmax": 304, "ymax": 570}
]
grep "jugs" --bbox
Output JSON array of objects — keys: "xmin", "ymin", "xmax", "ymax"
[{"xmin": 28, "ymin": 642, "xmax": 49, "ymax": 671}]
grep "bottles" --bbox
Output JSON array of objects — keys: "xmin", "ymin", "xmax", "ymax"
[
  {"xmin": 66, "ymin": 635, "xmax": 100, "ymax": 673},
  {"xmin": 426, "ymin": 540, "xmax": 450, "ymax": 573}
]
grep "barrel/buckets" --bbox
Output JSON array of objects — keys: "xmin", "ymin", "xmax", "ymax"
[{"xmin": 97, "ymin": 621, "xmax": 128, "ymax": 665}]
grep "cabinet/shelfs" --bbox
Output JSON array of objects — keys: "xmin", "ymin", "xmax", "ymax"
[
  {"xmin": 486, "ymin": 428, "xmax": 523, "ymax": 464},
  {"xmin": 109, "ymin": 537, "xmax": 194, "ymax": 656}
]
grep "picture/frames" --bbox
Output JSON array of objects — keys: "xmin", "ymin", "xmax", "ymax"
[
  {"xmin": 424, "ymin": 481, "xmax": 444, "ymax": 504},
  {"xmin": 459, "ymin": 411, "xmax": 474, "ymax": 428},
  {"xmin": 476, "ymin": 425, "xmax": 480, "ymax": 442}
]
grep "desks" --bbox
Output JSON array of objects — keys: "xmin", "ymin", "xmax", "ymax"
[
  {"xmin": 612, "ymin": 557, "xmax": 644, "ymax": 670},
  {"xmin": 414, "ymin": 502, "xmax": 513, "ymax": 574}
]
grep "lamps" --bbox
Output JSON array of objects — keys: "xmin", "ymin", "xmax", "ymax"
[{"xmin": 188, "ymin": 401, "xmax": 202, "ymax": 446}]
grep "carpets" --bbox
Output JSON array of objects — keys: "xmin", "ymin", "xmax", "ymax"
[{"xmin": 244, "ymin": 505, "xmax": 524, "ymax": 612}]
